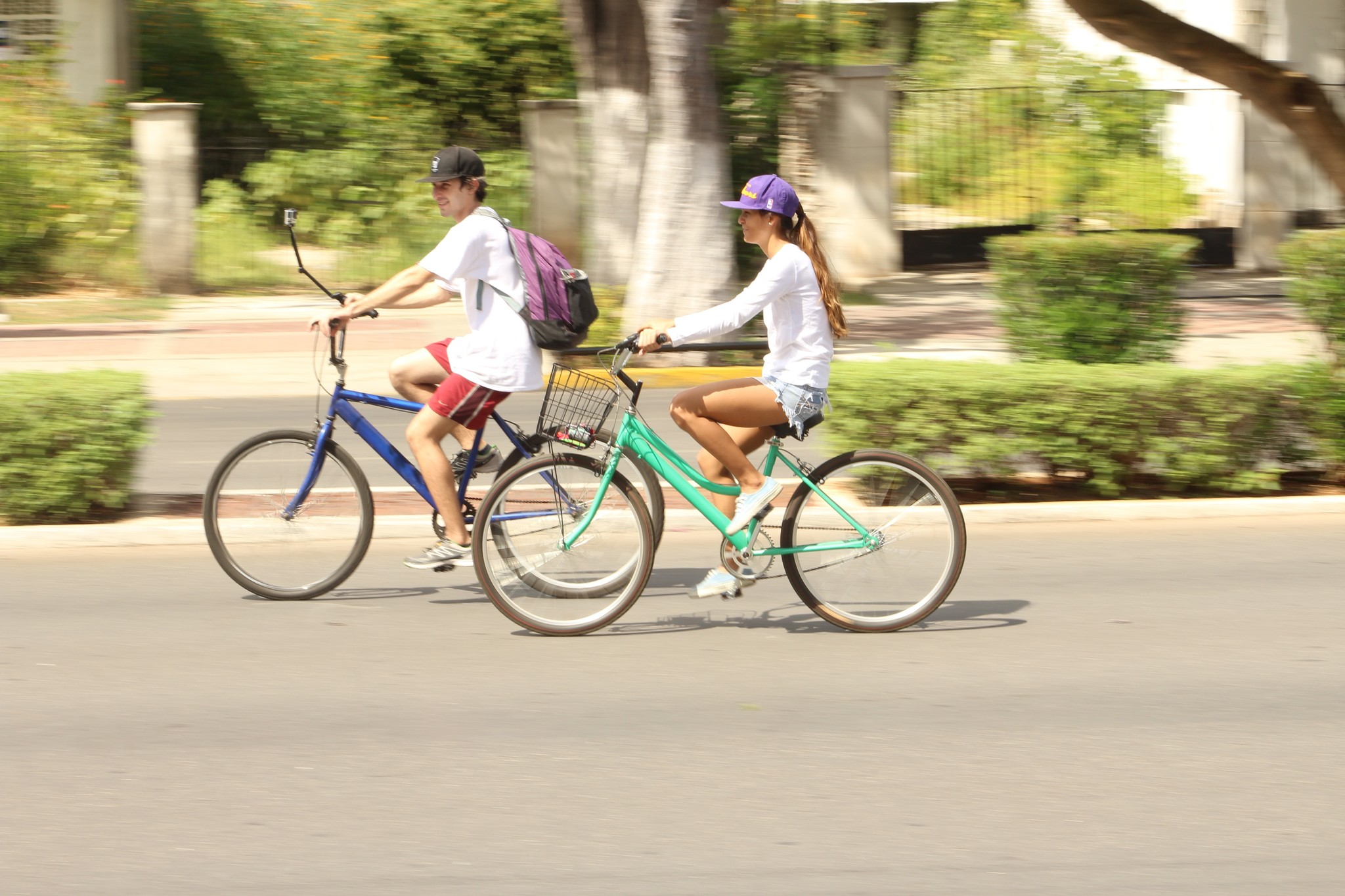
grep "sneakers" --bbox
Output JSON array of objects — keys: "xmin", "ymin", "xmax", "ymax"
[
  {"xmin": 449, "ymin": 444, "xmax": 505, "ymax": 481},
  {"xmin": 725, "ymin": 475, "xmax": 784, "ymax": 537},
  {"xmin": 403, "ymin": 536, "xmax": 474, "ymax": 570},
  {"xmin": 687, "ymin": 566, "xmax": 755, "ymax": 599}
]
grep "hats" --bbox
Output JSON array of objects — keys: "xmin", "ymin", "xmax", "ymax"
[
  {"xmin": 720, "ymin": 174, "xmax": 799, "ymax": 219},
  {"xmin": 414, "ymin": 144, "xmax": 485, "ymax": 183}
]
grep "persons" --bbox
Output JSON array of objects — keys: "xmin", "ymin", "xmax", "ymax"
[
  {"xmin": 313, "ymin": 142, "xmax": 545, "ymax": 571},
  {"xmin": 638, "ymin": 173, "xmax": 849, "ymax": 599}
]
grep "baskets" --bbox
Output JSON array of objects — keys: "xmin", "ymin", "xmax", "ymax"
[{"xmin": 536, "ymin": 362, "xmax": 621, "ymax": 452}]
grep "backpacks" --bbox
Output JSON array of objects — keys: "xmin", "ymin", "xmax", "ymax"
[{"xmin": 471, "ymin": 206, "xmax": 599, "ymax": 351}]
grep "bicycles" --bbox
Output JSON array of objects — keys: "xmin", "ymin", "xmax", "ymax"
[
  {"xmin": 200, "ymin": 292, "xmax": 665, "ymax": 605},
  {"xmin": 470, "ymin": 327, "xmax": 969, "ymax": 637}
]
